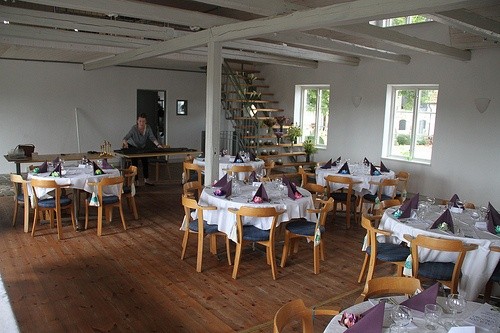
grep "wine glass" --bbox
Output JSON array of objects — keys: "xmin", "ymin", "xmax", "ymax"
[
  {"xmin": 446, "ymin": 293, "xmax": 467, "ymax": 326},
  {"xmin": 416, "ymin": 195, "xmax": 488, "ymax": 234},
  {"xmin": 390, "ymin": 304, "xmax": 413, "ymax": 333}
]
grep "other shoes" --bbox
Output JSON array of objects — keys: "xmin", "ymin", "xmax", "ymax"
[
  {"xmin": 133, "ymin": 180, "xmax": 140, "ymax": 187},
  {"xmin": 144, "ymin": 178, "xmax": 153, "ymax": 185}
]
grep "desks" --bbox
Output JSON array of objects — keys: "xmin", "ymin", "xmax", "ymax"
[
  {"xmin": 193, "ymin": 156, "xmax": 266, "ymax": 180},
  {"xmin": 115, "ymin": 150, "xmax": 205, "ymax": 185},
  {"xmin": 26, "ymin": 161, "xmax": 124, "ymax": 232},
  {"xmin": 323, "ymin": 295, "xmax": 500, "ymax": 333},
  {"xmin": 194, "ymin": 182, "xmax": 318, "ymax": 243},
  {"xmin": 315, "ymin": 164, "xmax": 396, "ymax": 213},
  {"xmin": 16, "ymin": 151, "xmax": 116, "ymax": 195},
  {"xmin": 362, "ymin": 201, "xmax": 500, "ymax": 301}
]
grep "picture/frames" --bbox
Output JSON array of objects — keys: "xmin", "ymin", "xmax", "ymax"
[{"xmin": 176, "ymin": 100, "xmax": 188, "ymax": 116}]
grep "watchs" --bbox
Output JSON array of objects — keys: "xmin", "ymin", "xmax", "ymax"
[{"xmin": 157, "ymin": 144, "xmax": 160, "ymax": 147}]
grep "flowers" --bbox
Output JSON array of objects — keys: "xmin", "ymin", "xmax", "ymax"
[{"xmin": 273, "ymin": 114, "xmax": 287, "ymax": 138}]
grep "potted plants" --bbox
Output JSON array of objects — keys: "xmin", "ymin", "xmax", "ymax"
[{"xmin": 288, "ymin": 123, "xmax": 315, "ymax": 163}]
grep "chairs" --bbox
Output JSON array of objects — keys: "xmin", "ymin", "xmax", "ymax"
[
  {"xmin": 10, "ymin": 165, "xmax": 138, "ymax": 240},
  {"xmin": 181, "ymin": 161, "xmax": 500, "ymax": 333}
]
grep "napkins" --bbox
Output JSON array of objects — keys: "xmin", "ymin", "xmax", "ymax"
[
  {"xmin": 430, "ymin": 208, "xmax": 454, "ymax": 234},
  {"xmin": 392, "ymin": 199, "xmax": 412, "ymax": 219},
  {"xmin": 218, "ymin": 179, "xmax": 232, "ymax": 198},
  {"xmin": 344, "ymin": 300, "xmax": 385, "ymax": 333},
  {"xmin": 288, "ymin": 181, "xmax": 304, "ymax": 201},
  {"xmin": 252, "ymin": 183, "xmax": 269, "ymax": 203},
  {"xmin": 213, "ymin": 173, "xmax": 227, "ymax": 187},
  {"xmin": 399, "ymin": 281, "xmax": 439, "ymax": 312},
  {"xmin": 411, "ymin": 192, "xmax": 419, "ymax": 209}
]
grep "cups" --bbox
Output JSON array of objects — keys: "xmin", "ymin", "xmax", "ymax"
[
  {"xmin": 424, "ymin": 303, "xmax": 443, "ymax": 330},
  {"xmin": 198, "ymin": 149, "xmax": 383, "ymax": 201}
]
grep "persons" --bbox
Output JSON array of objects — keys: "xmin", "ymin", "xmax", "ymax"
[{"xmin": 122, "ymin": 112, "xmax": 163, "ymax": 186}]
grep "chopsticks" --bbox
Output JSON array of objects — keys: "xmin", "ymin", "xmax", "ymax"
[{"xmin": 388, "ymin": 298, "xmax": 419, "ymax": 327}]
